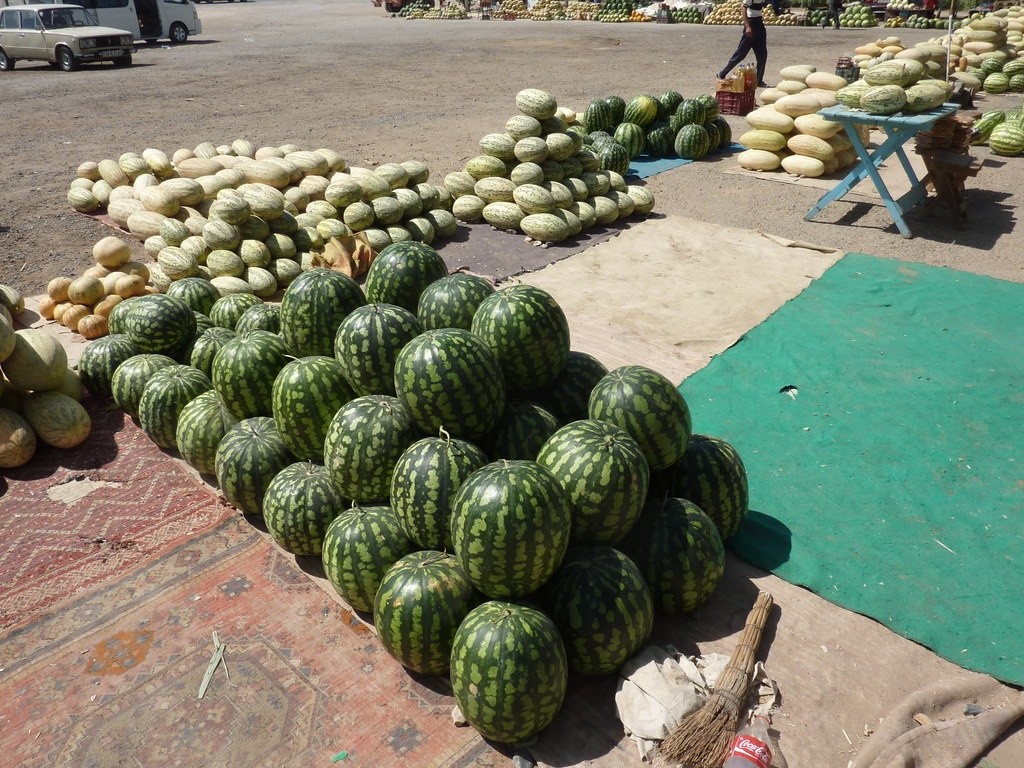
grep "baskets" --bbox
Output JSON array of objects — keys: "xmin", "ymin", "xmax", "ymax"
[
  {"xmin": 716, "ymin": 90, "xmax": 755, "ymax": 115},
  {"xmin": 657, "ymin": 10, "xmax": 675, "ymax": 23},
  {"xmin": 836, "ymin": 67, "xmax": 860, "ymax": 83}
]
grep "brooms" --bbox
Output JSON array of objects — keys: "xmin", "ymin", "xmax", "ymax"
[{"xmin": 647, "ymin": 589, "xmax": 775, "ymax": 767}]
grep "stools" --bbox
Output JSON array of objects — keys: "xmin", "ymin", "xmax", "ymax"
[{"xmin": 914, "ymin": 147, "xmax": 986, "ymax": 232}]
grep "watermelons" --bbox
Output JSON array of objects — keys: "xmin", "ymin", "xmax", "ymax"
[
  {"xmin": 598, "ymin": 0, "xmax": 702, "ymax": 23},
  {"xmin": 1, "ymin": 90, "xmax": 731, "ymax": 743}
]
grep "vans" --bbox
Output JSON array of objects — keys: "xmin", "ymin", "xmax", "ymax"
[{"xmin": 28, "ymin": 0, "xmax": 202, "ymax": 44}]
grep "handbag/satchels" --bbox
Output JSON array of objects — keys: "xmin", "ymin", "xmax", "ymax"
[{"xmin": 749, "ymin": 3, "xmax": 762, "ymax": 10}]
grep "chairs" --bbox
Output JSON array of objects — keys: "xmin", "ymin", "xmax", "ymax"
[
  {"xmin": 22, "ymin": 18, "xmax": 34, "ymax": 27},
  {"xmin": 54, "ymin": 15, "xmax": 66, "ymax": 26}
]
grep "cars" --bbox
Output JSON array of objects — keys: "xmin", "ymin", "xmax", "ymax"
[{"xmin": 0, "ymin": 3, "xmax": 138, "ymax": 71}]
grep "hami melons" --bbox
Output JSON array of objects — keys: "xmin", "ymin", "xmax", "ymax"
[
  {"xmin": 494, "ymin": 0, "xmax": 599, "ymax": 20},
  {"xmin": 703, "ymin": 0, "xmax": 1024, "ymax": 177}
]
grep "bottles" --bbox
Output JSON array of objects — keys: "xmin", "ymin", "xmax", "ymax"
[
  {"xmin": 766, "ymin": 728, "xmax": 788, "ymax": 768},
  {"xmin": 722, "ymin": 713, "xmax": 773, "ymax": 768},
  {"xmin": 731, "ymin": 62, "xmax": 757, "ymax": 92}
]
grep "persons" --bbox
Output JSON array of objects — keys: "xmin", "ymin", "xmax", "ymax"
[
  {"xmin": 715, "ymin": 0, "xmax": 768, "ymax": 88},
  {"xmin": 817, "ymin": 0, "xmax": 846, "ymax": 30}
]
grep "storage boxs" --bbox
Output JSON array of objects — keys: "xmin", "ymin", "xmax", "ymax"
[
  {"xmin": 835, "ymin": 66, "xmax": 861, "ymax": 83},
  {"xmin": 657, "ymin": 10, "xmax": 675, "ymax": 24},
  {"xmin": 715, "ymin": 90, "xmax": 756, "ymax": 116}
]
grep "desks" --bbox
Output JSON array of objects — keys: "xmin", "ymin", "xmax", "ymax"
[
  {"xmin": 883, "ymin": 8, "xmax": 933, "ymax": 24},
  {"xmin": 804, "ymin": 104, "xmax": 961, "ymax": 237}
]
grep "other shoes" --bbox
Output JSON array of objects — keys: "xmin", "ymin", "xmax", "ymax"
[
  {"xmin": 716, "ymin": 74, "xmax": 725, "ymax": 79},
  {"xmin": 757, "ymin": 82, "xmax": 767, "ymax": 87}
]
